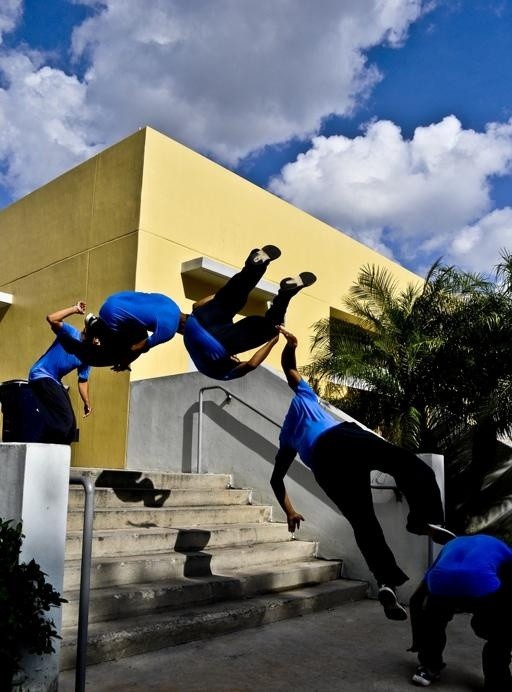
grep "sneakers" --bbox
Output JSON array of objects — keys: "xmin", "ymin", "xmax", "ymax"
[
  {"xmin": 405, "ymin": 515, "xmax": 457, "ymax": 546},
  {"xmin": 84, "ymin": 311, "xmax": 99, "ymax": 333},
  {"xmin": 410, "ymin": 663, "xmax": 440, "ymax": 689},
  {"xmin": 246, "ymin": 245, "xmax": 282, "ymax": 268},
  {"xmin": 376, "ymin": 584, "xmax": 408, "ymax": 621},
  {"xmin": 278, "ymin": 271, "xmax": 317, "ymax": 294}
]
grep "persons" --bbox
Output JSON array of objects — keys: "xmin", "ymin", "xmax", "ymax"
[
  {"xmin": 269, "ymin": 324, "xmax": 457, "ymax": 620},
  {"xmin": 185, "ymin": 245, "xmax": 317, "ymax": 379},
  {"xmin": 409, "ymin": 533, "xmax": 511, "ymax": 691},
  {"xmin": 89, "ymin": 292, "xmax": 191, "ymax": 372},
  {"xmin": 1, "ymin": 301, "xmax": 100, "ymax": 442}
]
